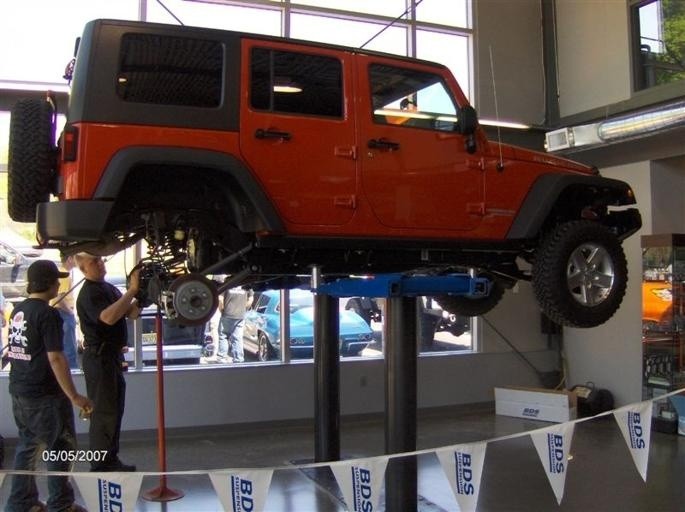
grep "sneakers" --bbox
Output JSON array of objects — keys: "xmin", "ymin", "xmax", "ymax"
[
  {"xmin": 62, "ymin": 502, "xmax": 87, "ymax": 512},
  {"xmin": 29, "ymin": 501, "xmax": 45, "ymax": 512}
]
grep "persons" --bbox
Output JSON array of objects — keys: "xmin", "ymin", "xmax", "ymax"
[
  {"xmin": 214, "ymin": 285, "xmax": 255, "ymax": 364},
  {"xmin": 3, "ymin": 261, "xmax": 98, "ymax": 511},
  {"xmin": 49, "ymin": 250, "xmax": 80, "ymax": 371},
  {"xmin": 1, "ymin": 253, "xmax": 25, "ymax": 370},
  {"xmin": 74, "ymin": 249, "xmax": 147, "ymax": 473}
]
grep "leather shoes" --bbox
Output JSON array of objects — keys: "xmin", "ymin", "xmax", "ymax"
[
  {"xmin": 90, "ymin": 453, "xmax": 100, "ymax": 471},
  {"xmin": 100, "ymin": 456, "xmax": 136, "ymax": 472}
]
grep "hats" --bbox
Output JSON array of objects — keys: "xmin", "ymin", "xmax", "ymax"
[{"xmin": 28, "ymin": 260, "xmax": 70, "ymax": 281}]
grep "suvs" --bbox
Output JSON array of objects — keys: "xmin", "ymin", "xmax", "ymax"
[{"xmin": 7, "ymin": 18, "xmax": 644, "ymax": 331}]
